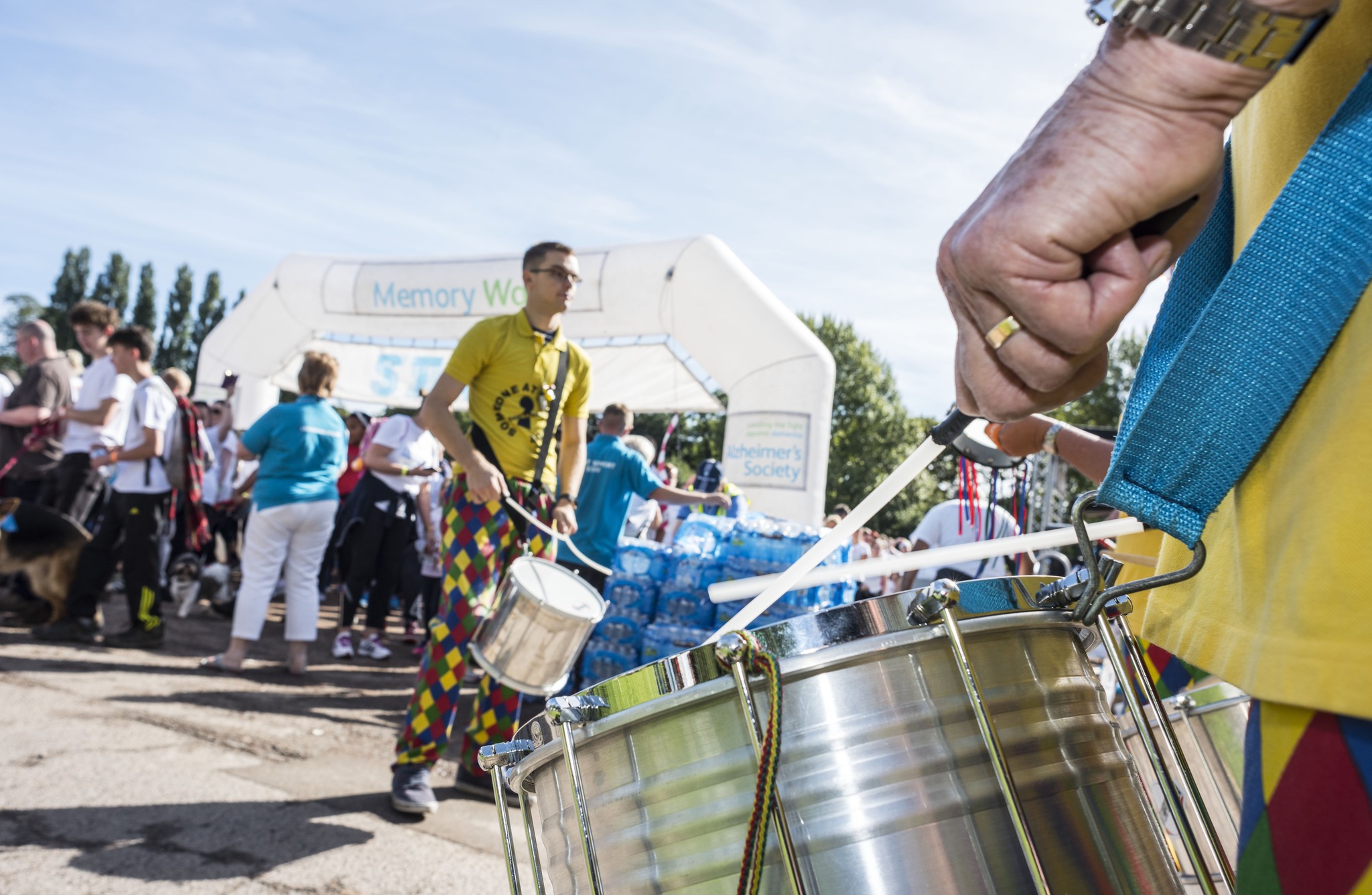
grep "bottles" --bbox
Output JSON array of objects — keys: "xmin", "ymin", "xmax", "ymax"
[
  {"xmin": 90, "ymin": 445, "xmax": 120, "ymax": 484},
  {"xmin": 557, "ymin": 514, "xmax": 858, "ymax": 699}
]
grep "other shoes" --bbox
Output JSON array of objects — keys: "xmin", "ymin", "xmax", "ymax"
[
  {"xmin": 102, "ymin": 626, "xmax": 166, "ymax": 648},
  {"xmin": 402, "ymin": 621, "xmax": 420, "ymax": 645},
  {"xmin": 32, "ymin": 614, "xmax": 102, "ymax": 642},
  {"xmin": 357, "ymin": 632, "xmax": 392, "ymax": 660},
  {"xmin": 330, "ymin": 630, "xmax": 355, "ymax": 659},
  {"xmin": 392, "ymin": 764, "xmax": 438, "ymax": 814},
  {"xmin": 455, "ymin": 765, "xmax": 536, "ymax": 807}
]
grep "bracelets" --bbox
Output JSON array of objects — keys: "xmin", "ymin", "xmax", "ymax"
[
  {"xmin": 558, "ymin": 493, "xmax": 578, "ymax": 510},
  {"xmin": 241, "ymin": 492, "xmax": 251, "ymax": 500},
  {"xmin": 401, "ymin": 468, "xmax": 408, "ymax": 475},
  {"xmin": 1086, "ymin": 0, "xmax": 1333, "ymax": 72}
]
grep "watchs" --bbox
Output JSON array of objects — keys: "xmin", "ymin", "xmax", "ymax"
[{"xmin": 1042, "ymin": 421, "xmax": 1067, "ymax": 456}]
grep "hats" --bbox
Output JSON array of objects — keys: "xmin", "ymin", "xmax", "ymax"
[{"xmin": 691, "ymin": 459, "xmax": 725, "ymax": 493}]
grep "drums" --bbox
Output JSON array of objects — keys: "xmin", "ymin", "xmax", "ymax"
[
  {"xmin": 1115, "ymin": 683, "xmax": 1252, "ymax": 895},
  {"xmin": 487, "ymin": 570, "xmax": 1190, "ymax": 895},
  {"xmin": 467, "ymin": 555, "xmax": 608, "ymax": 697},
  {"xmin": 29, "ymin": 449, "xmax": 115, "ymax": 546}
]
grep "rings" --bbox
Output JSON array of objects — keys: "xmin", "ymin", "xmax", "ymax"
[{"xmin": 984, "ymin": 315, "xmax": 1022, "ymax": 350}]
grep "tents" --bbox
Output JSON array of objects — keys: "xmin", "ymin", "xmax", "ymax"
[{"xmin": 189, "ymin": 233, "xmax": 836, "ymax": 530}]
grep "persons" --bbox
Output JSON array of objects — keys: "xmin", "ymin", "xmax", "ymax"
[
  {"xmin": 897, "ymin": 491, "xmax": 1038, "ymax": 591},
  {"xmin": 936, "ymin": 0, "xmax": 1372, "ymax": 895},
  {"xmin": 822, "ymin": 503, "xmax": 910, "ymax": 603},
  {"xmin": 556, "ymin": 403, "xmax": 733, "ymax": 597},
  {"xmin": 0, "ymin": 298, "xmax": 454, "ymax": 676},
  {"xmin": 619, "ymin": 435, "xmax": 670, "ymax": 543},
  {"xmin": 389, "ymin": 241, "xmax": 594, "ymax": 815}
]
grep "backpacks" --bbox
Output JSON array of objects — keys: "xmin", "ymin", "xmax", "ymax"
[{"xmin": 134, "ymin": 383, "xmax": 215, "ymax": 496}]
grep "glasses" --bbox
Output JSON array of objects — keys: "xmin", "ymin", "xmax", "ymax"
[
  {"xmin": 211, "ymin": 407, "xmax": 221, "ymax": 414},
  {"xmin": 530, "ymin": 269, "xmax": 581, "ymax": 285}
]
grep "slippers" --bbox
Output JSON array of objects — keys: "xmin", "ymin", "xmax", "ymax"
[
  {"xmin": 200, "ymin": 653, "xmax": 241, "ymax": 673},
  {"xmin": 284, "ymin": 659, "xmax": 308, "ymax": 677}
]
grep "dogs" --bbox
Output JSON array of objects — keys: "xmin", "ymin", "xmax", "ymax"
[
  {"xmin": 166, "ymin": 549, "xmax": 241, "ymax": 622},
  {"xmin": 0, "ymin": 493, "xmax": 108, "ymax": 645}
]
